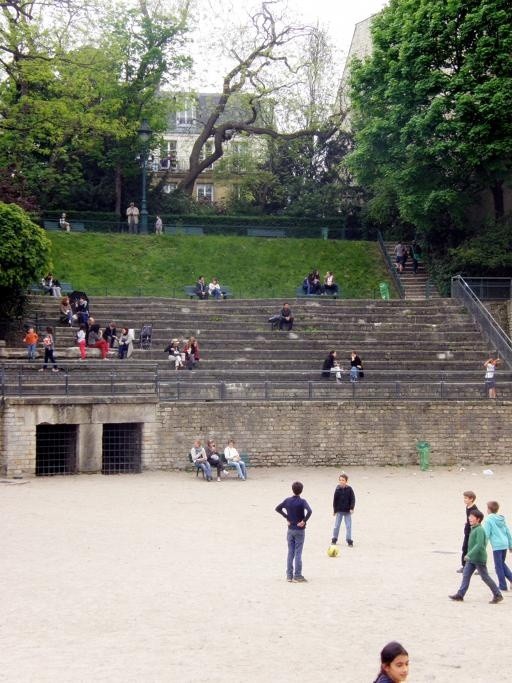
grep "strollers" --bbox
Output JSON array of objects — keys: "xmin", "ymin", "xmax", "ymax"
[{"xmin": 138, "ymin": 321, "xmax": 154, "ymax": 349}]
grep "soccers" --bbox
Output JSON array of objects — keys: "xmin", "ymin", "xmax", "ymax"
[{"xmin": 327, "ymin": 545, "xmax": 340, "ymax": 556}]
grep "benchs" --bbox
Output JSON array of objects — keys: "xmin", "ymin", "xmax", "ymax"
[
  {"xmin": 162, "ymin": 224, "xmax": 204, "ymax": 236},
  {"xmin": 244, "ymin": 228, "xmax": 287, "ymax": 239},
  {"xmin": 189, "ymin": 454, "xmax": 250, "ymax": 478},
  {"xmin": 40, "ymin": 221, "xmax": 85, "ymax": 233}
]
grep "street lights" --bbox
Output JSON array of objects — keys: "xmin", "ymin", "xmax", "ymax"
[{"xmin": 136, "ymin": 117, "xmax": 153, "ymax": 235}]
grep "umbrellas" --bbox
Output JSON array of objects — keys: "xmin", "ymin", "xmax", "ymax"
[{"xmin": 69, "ymin": 290, "xmax": 90, "ymax": 312}]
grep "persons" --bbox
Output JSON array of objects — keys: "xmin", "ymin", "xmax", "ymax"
[
  {"xmin": 190, "ymin": 439, "xmax": 214, "ymax": 481},
  {"xmin": 393, "ymin": 240, "xmax": 405, "ymax": 271},
  {"xmin": 301, "ymin": 268, "xmax": 338, "ymax": 297},
  {"xmin": 57, "ymin": 295, "xmax": 75, "ymax": 328},
  {"xmin": 182, "ymin": 336, "xmax": 200, "ymax": 370},
  {"xmin": 454, "ymin": 490, "xmax": 487, "ymax": 575},
  {"xmin": 331, "ymin": 474, "xmax": 355, "ymax": 546},
  {"xmin": 79, "ymin": 316, "xmax": 96, "ymax": 332},
  {"xmin": 447, "ymin": 508, "xmax": 505, "ymax": 604},
  {"xmin": 117, "ymin": 326, "xmax": 134, "ymax": 360},
  {"xmin": 482, "ymin": 499, "xmax": 512, "ymax": 592},
  {"xmin": 102, "ymin": 321, "xmax": 118, "ymax": 348},
  {"xmin": 153, "ymin": 214, "xmax": 164, "ymax": 235},
  {"xmin": 59, "ymin": 212, "xmax": 70, "ymax": 232},
  {"xmin": 275, "ymin": 481, "xmax": 313, "ymax": 583},
  {"xmin": 195, "ymin": 275, "xmax": 209, "ymax": 300},
  {"xmin": 164, "ymin": 337, "xmax": 187, "ymax": 370},
  {"xmin": 206, "ymin": 437, "xmax": 229, "ymax": 482},
  {"xmin": 36, "ymin": 325, "xmax": 60, "ymax": 372},
  {"xmin": 41, "ymin": 272, "xmax": 53, "ymax": 296},
  {"xmin": 278, "ymin": 301, "xmax": 294, "ymax": 330},
  {"xmin": 208, "ymin": 275, "xmax": 223, "ymax": 299},
  {"xmin": 22, "ymin": 324, "xmax": 39, "ymax": 364},
  {"xmin": 409, "ymin": 239, "xmax": 422, "ymax": 273},
  {"xmin": 74, "ymin": 294, "xmax": 91, "ymax": 324},
  {"xmin": 49, "ymin": 273, "xmax": 63, "ymax": 299},
  {"xmin": 86, "ymin": 323, "xmax": 111, "ymax": 361},
  {"xmin": 483, "ymin": 357, "xmax": 497, "ymax": 400},
  {"xmin": 320, "ymin": 349, "xmax": 345, "ymax": 384},
  {"xmin": 371, "ymin": 641, "xmax": 411, "ymax": 682},
  {"xmin": 126, "ymin": 201, "xmax": 140, "ymax": 234},
  {"xmin": 349, "ymin": 349, "xmax": 364, "ymax": 384},
  {"xmin": 224, "ymin": 439, "xmax": 248, "ymax": 480},
  {"xmin": 75, "ymin": 322, "xmax": 88, "ymax": 359}
]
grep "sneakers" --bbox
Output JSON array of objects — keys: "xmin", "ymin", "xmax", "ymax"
[
  {"xmin": 348, "ymin": 540, "xmax": 353, "ymax": 548},
  {"xmin": 448, "ymin": 594, "xmax": 464, "ymax": 602},
  {"xmin": 287, "ymin": 576, "xmax": 309, "ymax": 583},
  {"xmin": 498, "ymin": 587, "xmax": 508, "ymax": 591},
  {"xmin": 174, "ymin": 363, "xmax": 199, "ymax": 370},
  {"xmin": 37, "ymin": 368, "xmax": 60, "ymax": 373},
  {"xmin": 331, "ymin": 539, "xmax": 336, "ymax": 545},
  {"xmin": 455, "ymin": 567, "xmax": 480, "ymax": 575},
  {"xmin": 488, "ymin": 593, "xmax": 504, "ymax": 604},
  {"xmin": 78, "ymin": 351, "xmax": 127, "ymax": 362},
  {"xmin": 207, "ymin": 469, "xmax": 247, "ymax": 482}
]
